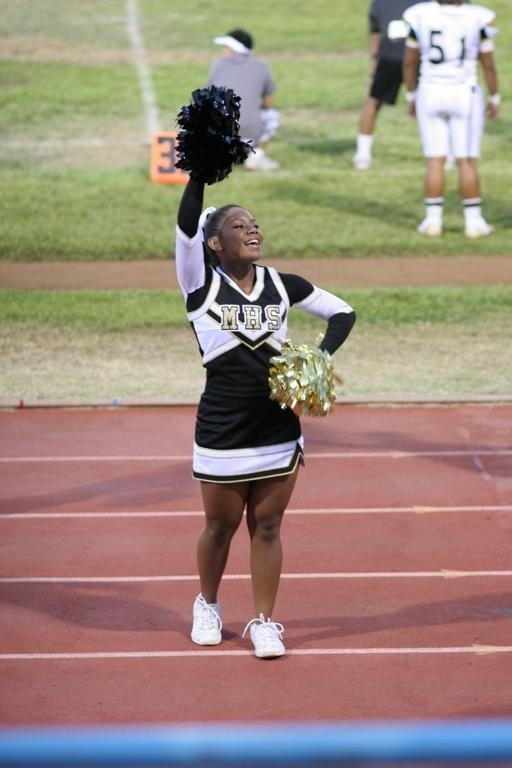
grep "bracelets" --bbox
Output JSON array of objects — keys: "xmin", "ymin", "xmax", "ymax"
[
  {"xmin": 404, "ymin": 91, "xmax": 416, "ymax": 102},
  {"xmin": 487, "ymin": 94, "xmax": 500, "ymax": 105}
]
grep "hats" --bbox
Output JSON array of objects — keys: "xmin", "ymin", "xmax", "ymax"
[{"xmin": 213, "ymin": 35, "xmax": 251, "ymax": 56}]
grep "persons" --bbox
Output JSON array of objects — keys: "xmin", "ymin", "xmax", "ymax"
[
  {"xmin": 400, "ymin": 0, "xmax": 502, "ymax": 240},
  {"xmin": 204, "ymin": 28, "xmax": 282, "ymax": 171},
  {"xmin": 172, "ymin": 173, "xmax": 358, "ymax": 663},
  {"xmin": 353, "ymin": 0, "xmax": 471, "ymax": 171}
]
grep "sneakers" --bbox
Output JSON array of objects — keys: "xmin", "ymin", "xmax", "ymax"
[
  {"xmin": 417, "ymin": 218, "xmax": 442, "ymax": 237},
  {"xmin": 244, "ymin": 154, "xmax": 281, "ymax": 171},
  {"xmin": 464, "ymin": 216, "xmax": 495, "ymax": 240},
  {"xmin": 353, "ymin": 153, "xmax": 372, "ymax": 170},
  {"xmin": 191, "ymin": 594, "xmax": 223, "ymax": 645},
  {"xmin": 241, "ymin": 613, "xmax": 285, "ymax": 658}
]
